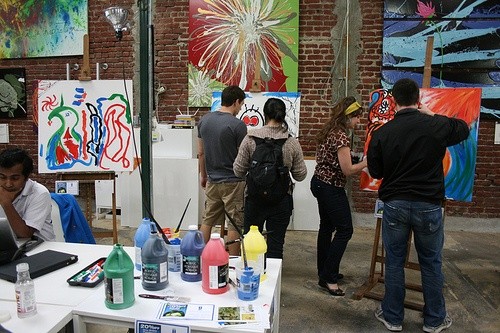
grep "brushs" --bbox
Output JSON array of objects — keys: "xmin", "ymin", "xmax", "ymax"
[{"xmin": 139, "ymin": 294, "xmax": 190, "ymax": 302}]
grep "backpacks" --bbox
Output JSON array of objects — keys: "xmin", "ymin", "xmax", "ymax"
[{"xmin": 246, "ymin": 134, "xmax": 293, "ymax": 196}]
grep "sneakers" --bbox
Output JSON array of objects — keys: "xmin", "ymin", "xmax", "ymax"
[
  {"xmin": 374, "ymin": 304, "xmax": 402, "ymax": 331},
  {"xmin": 423, "ymin": 312, "xmax": 452, "ymax": 333}
]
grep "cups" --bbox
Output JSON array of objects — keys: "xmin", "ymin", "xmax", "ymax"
[{"xmin": 234, "ymin": 260, "xmax": 260, "ymax": 301}]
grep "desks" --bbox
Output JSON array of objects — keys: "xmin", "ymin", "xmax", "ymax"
[
  {"xmin": 0, "ymin": 242, "xmax": 282, "ymax": 333},
  {"xmin": 95, "ymin": 125, "xmax": 319, "ymax": 231}
]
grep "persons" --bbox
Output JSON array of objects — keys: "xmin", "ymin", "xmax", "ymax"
[
  {"xmin": 367, "ymin": 79, "xmax": 470, "ymax": 333},
  {"xmin": 0, "ymin": 148, "xmax": 57, "ymax": 242},
  {"xmin": 197, "ymin": 86, "xmax": 248, "ymax": 256},
  {"xmin": 310, "ymin": 96, "xmax": 369, "ymax": 296},
  {"xmin": 232, "ymin": 98, "xmax": 307, "ymax": 259}
]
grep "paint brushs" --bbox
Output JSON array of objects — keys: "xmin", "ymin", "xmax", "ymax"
[
  {"xmin": 229, "ymin": 257, "xmax": 238, "ymax": 258},
  {"xmin": 143, "ymin": 203, "xmax": 171, "ymax": 244},
  {"xmin": 222, "ymin": 239, "xmax": 241, "ymax": 245},
  {"xmin": 242, "ymin": 239, "xmax": 248, "ymax": 270},
  {"xmin": 243, "ymin": 231, "xmax": 274, "ymax": 237},
  {"xmin": 223, "ymin": 209, "xmax": 244, "ymax": 238},
  {"xmin": 175, "ymin": 198, "xmax": 191, "ymax": 233},
  {"xmin": 296, "ymin": 136, "xmax": 306, "ymax": 138},
  {"xmin": 418, "ymin": 93, "xmax": 423, "ymax": 105}
]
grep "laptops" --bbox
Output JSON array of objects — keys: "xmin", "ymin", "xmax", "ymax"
[
  {"xmin": 0, "ymin": 204, "xmax": 45, "ymax": 255},
  {"xmin": 0, "ymin": 249, "xmax": 78, "ymax": 283}
]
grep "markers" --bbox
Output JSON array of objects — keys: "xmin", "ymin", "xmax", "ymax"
[
  {"xmin": 74, "ymin": 260, "xmax": 105, "ymax": 283},
  {"xmin": 70, "ymin": 271, "xmax": 85, "ymax": 281}
]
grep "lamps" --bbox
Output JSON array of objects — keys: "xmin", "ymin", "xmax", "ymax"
[{"xmin": 104, "ymin": 6, "xmax": 133, "ymax": 39}]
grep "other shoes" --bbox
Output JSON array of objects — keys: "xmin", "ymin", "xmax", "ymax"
[
  {"xmin": 338, "ymin": 274, "xmax": 344, "ymax": 279},
  {"xmin": 318, "ymin": 278, "xmax": 345, "ymax": 296}
]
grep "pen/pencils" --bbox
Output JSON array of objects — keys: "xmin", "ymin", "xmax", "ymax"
[{"xmin": 229, "ymin": 278, "xmax": 237, "ymax": 288}]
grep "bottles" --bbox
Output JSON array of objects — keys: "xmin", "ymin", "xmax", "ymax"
[
  {"xmin": 200, "ymin": 233, "xmax": 230, "ymax": 295},
  {"xmin": 180, "ymin": 225, "xmax": 206, "ymax": 282},
  {"xmin": 14, "ymin": 263, "xmax": 37, "ymax": 318},
  {"xmin": 140, "ymin": 231, "xmax": 169, "ymax": 291},
  {"xmin": 240, "ymin": 226, "xmax": 268, "ymax": 281},
  {"xmin": 103, "ymin": 243, "xmax": 135, "ymax": 310},
  {"xmin": 134, "ymin": 217, "xmax": 157, "ymax": 271},
  {"xmin": 164, "ymin": 244, "xmax": 182, "ymax": 273}
]
